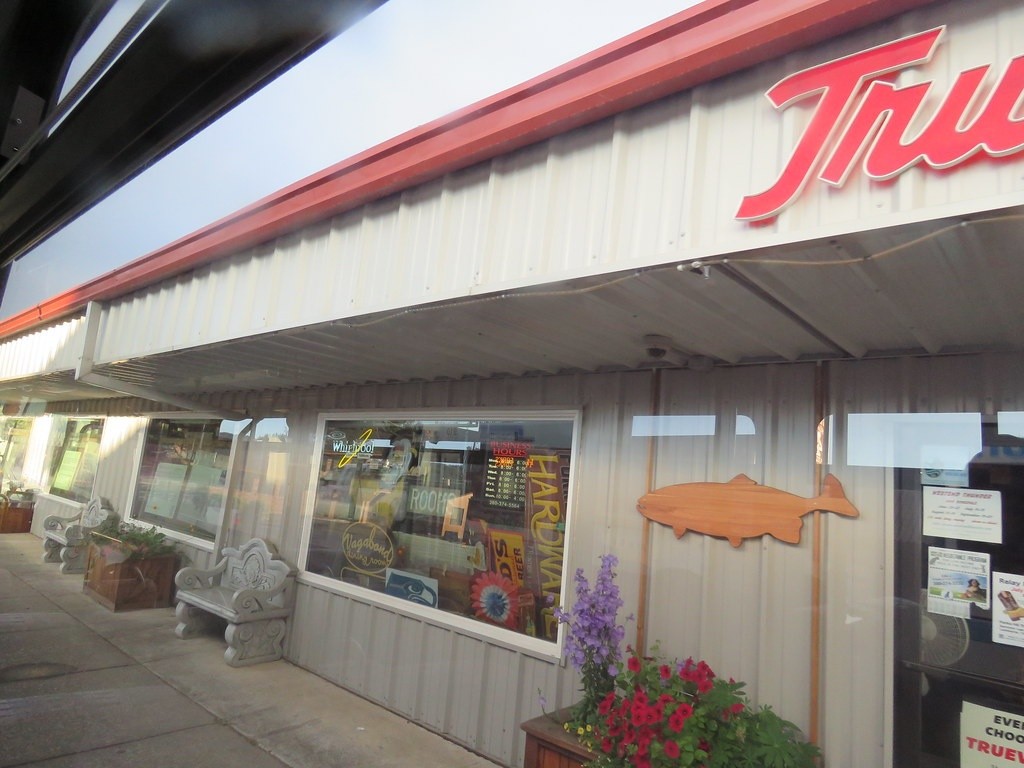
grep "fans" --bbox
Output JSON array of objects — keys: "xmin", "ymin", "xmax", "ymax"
[{"xmin": 920, "ymin": 587, "xmax": 969, "ymax": 667}]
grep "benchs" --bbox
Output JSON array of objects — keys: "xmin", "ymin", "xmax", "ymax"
[
  {"xmin": 42, "ymin": 495, "xmax": 119, "ymax": 574},
  {"xmin": 174, "ymin": 537, "xmax": 299, "ymax": 668}
]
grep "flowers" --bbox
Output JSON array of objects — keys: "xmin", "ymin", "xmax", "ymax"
[
  {"xmin": 537, "ymin": 552, "xmax": 822, "ymax": 768},
  {"xmin": 79, "ymin": 521, "xmax": 194, "ymax": 566}
]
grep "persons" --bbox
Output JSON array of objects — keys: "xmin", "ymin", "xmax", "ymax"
[
  {"xmin": 171, "ymin": 431, "xmax": 187, "ymax": 458},
  {"xmin": 320, "ymin": 458, "xmax": 333, "ymax": 481}
]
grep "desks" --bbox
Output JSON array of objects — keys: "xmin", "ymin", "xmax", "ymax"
[{"xmin": 393, "ymin": 530, "xmax": 477, "ymax": 614}]
